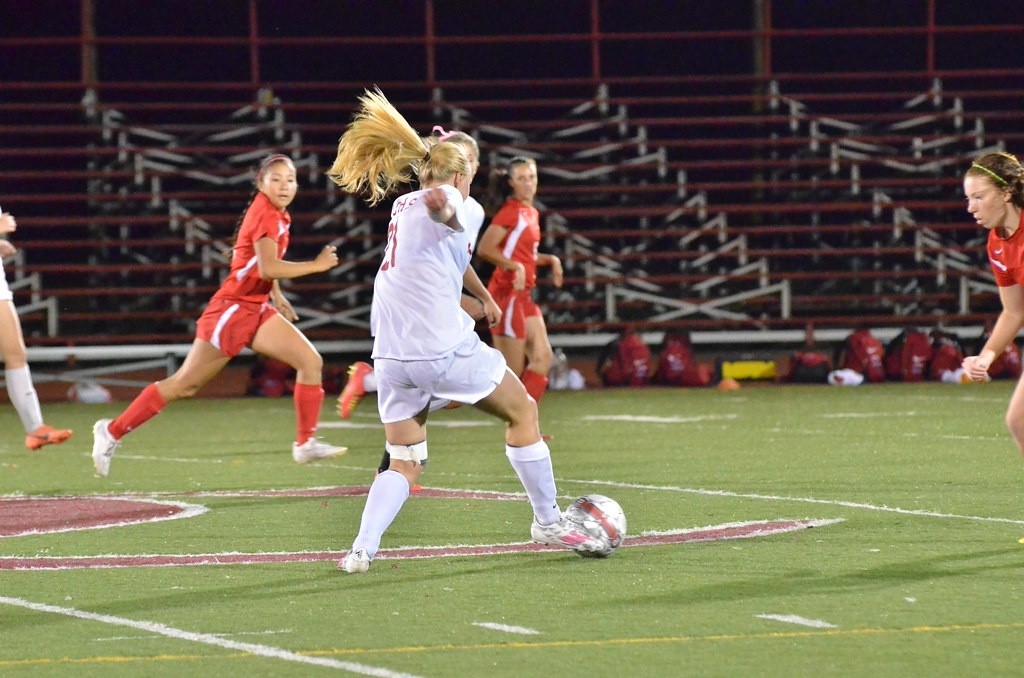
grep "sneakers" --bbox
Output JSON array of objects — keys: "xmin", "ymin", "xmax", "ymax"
[
  {"xmin": 531, "ymin": 514, "xmax": 604, "ymax": 553},
  {"xmin": 336, "ymin": 362, "xmax": 374, "ymax": 421},
  {"xmin": 337, "ymin": 548, "xmax": 371, "ymax": 574},
  {"xmin": 25, "ymin": 424, "xmax": 73, "ymax": 450},
  {"xmin": 91, "ymin": 418, "xmax": 123, "ymax": 477},
  {"xmin": 292, "ymin": 436, "xmax": 348, "ymax": 464}
]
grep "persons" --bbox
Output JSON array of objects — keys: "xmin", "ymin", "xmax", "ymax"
[
  {"xmin": 0, "ymin": 202, "xmax": 74, "ymax": 449},
  {"xmin": 327, "ymin": 83, "xmax": 607, "ymax": 573},
  {"xmin": 478, "ymin": 154, "xmax": 562, "ymax": 443},
  {"xmin": 336, "ymin": 127, "xmax": 485, "ymax": 418},
  {"xmin": 960, "ymin": 150, "xmax": 1024, "ymax": 461},
  {"xmin": 91, "ymin": 154, "xmax": 348, "ymax": 478}
]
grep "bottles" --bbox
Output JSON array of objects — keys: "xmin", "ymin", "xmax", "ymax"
[{"xmin": 548, "ymin": 348, "xmax": 568, "ymax": 391}]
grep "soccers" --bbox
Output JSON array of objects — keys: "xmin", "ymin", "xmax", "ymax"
[{"xmin": 558, "ymin": 493, "xmax": 629, "ymax": 560}]
